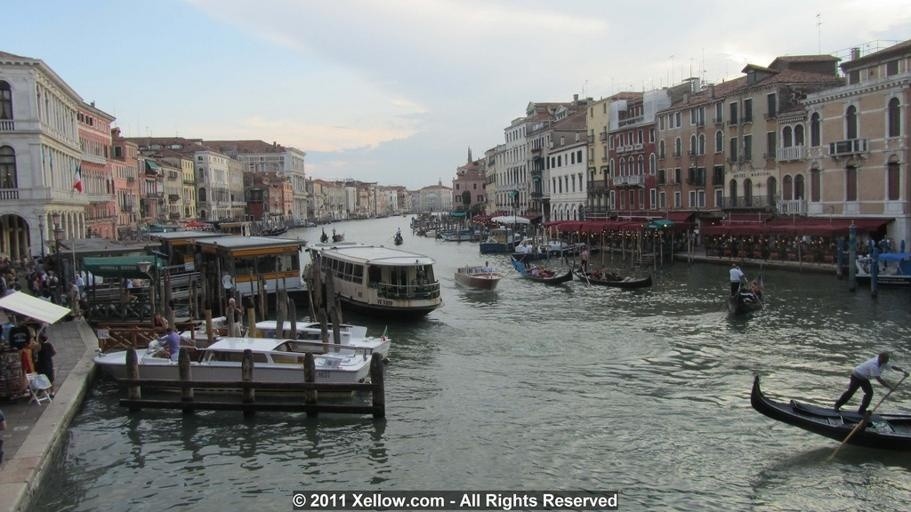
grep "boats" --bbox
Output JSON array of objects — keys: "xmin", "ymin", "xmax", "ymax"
[
  {"xmin": 93, "ymin": 316, "xmax": 392, "ymax": 399},
  {"xmin": 853, "ymin": 251, "xmax": 911, "ymax": 284},
  {"xmin": 751, "ymin": 376, "xmax": 911, "ymax": 451},
  {"xmin": 480, "ymin": 239, "xmax": 585, "ymax": 282},
  {"xmin": 726, "ymin": 275, "xmax": 764, "ymax": 312},
  {"xmin": 395, "ymin": 236, "xmax": 403, "ymax": 245},
  {"xmin": 59, "ymin": 225, "xmax": 314, "ymax": 313},
  {"xmin": 455, "ymin": 265, "xmax": 502, "ymax": 290},
  {"xmin": 333, "ymin": 235, "xmax": 344, "ymax": 241},
  {"xmin": 576, "ymin": 271, "xmax": 652, "ymax": 287},
  {"xmin": 304, "ymin": 241, "xmax": 442, "ymax": 315},
  {"xmin": 437, "ymin": 229, "xmax": 490, "ymax": 241},
  {"xmin": 320, "ymin": 232, "xmax": 328, "ymax": 242}
]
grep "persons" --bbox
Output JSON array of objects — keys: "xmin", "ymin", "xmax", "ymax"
[
  {"xmin": 122, "ymin": 277, "xmax": 133, "ymax": 290},
  {"xmin": 408, "ymin": 205, "xmax": 906, "ymax": 298},
  {"xmin": 224, "ymin": 298, "xmax": 243, "ymax": 337},
  {"xmin": 153, "ymin": 305, "xmax": 196, "ymax": 362},
  {"xmin": 394, "ymin": 226, "xmax": 402, "ymax": 240},
  {"xmin": 834, "ymin": 351, "xmax": 909, "ymax": 417},
  {"xmin": 133, "ymin": 279, "xmax": 144, "ymax": 288},
  {"xmin": 0, "ymin": 249, "xmax": 94, "ymax": 467}
]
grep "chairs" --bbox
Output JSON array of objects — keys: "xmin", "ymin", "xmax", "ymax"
[{"xmin": 25, "ymin": 372, "xmax": 52, "ymax": 405}]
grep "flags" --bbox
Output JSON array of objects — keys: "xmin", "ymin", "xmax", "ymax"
[{"xmin": 73, "ymin": 167, "xmax": 83, "ymax": 193}]
